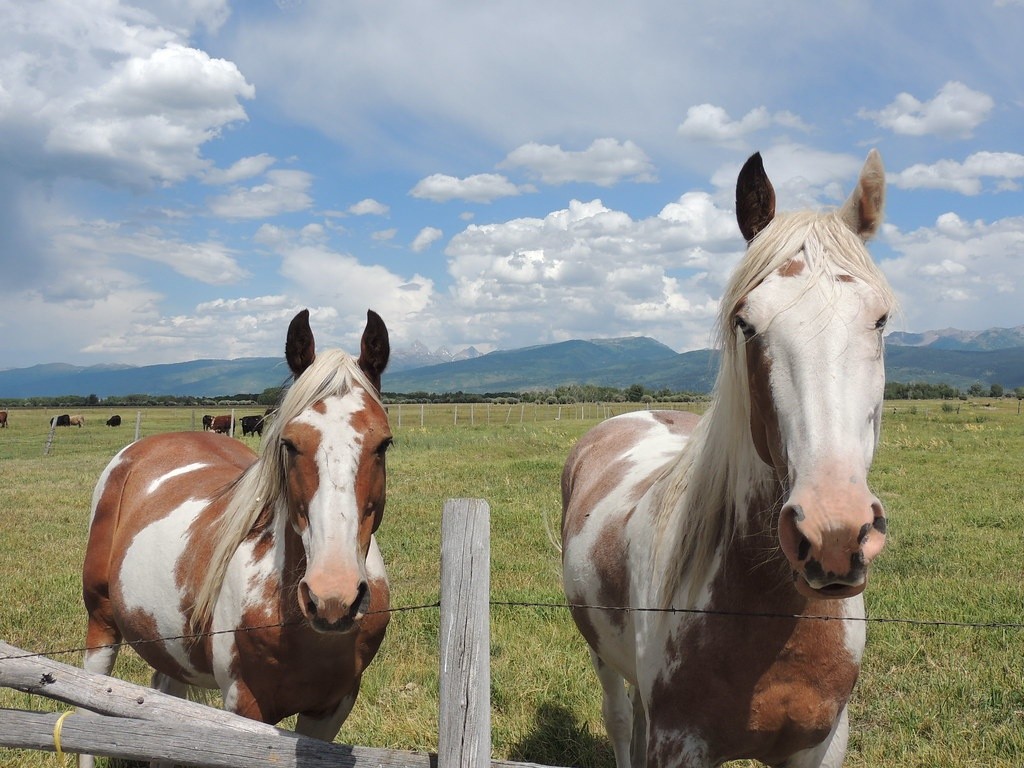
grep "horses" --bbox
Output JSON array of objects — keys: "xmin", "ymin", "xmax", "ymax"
[
  {"xmin": 83, "ymin": 309, "xmax": 392, "ymax": 741},
  {"xmin": 543, "ymin": 146, "xmax": 898, "ymax": 767}
]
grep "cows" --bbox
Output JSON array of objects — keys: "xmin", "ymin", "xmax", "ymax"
[
  {"xmin": 210, "ymin": 414, "xmax": 236, "ymax": 436},
  {"xmin": 0, "ymin": 411, "xmax": 9, "ymax": 428},
  {"xmin": 238, "ymin": 414, "xmax": 264, "ymax": 437},
  {"xmin": 50, "ymin": 414, "xmax": 70, "ymax": 427},
  {"xmin": 203, "ymin": 415, "xmax": 215, "ymax": 431},
  {"xmin": 106, "ymin": 414, "xmax": 122, "ymax": 427},
  {"xmin": 69, "ymin": 415, "xmax": 85, "ymax": 428},
  {"xmin": 264, "ymin": 409, "xmax": 279, "ymax": 419}
]
